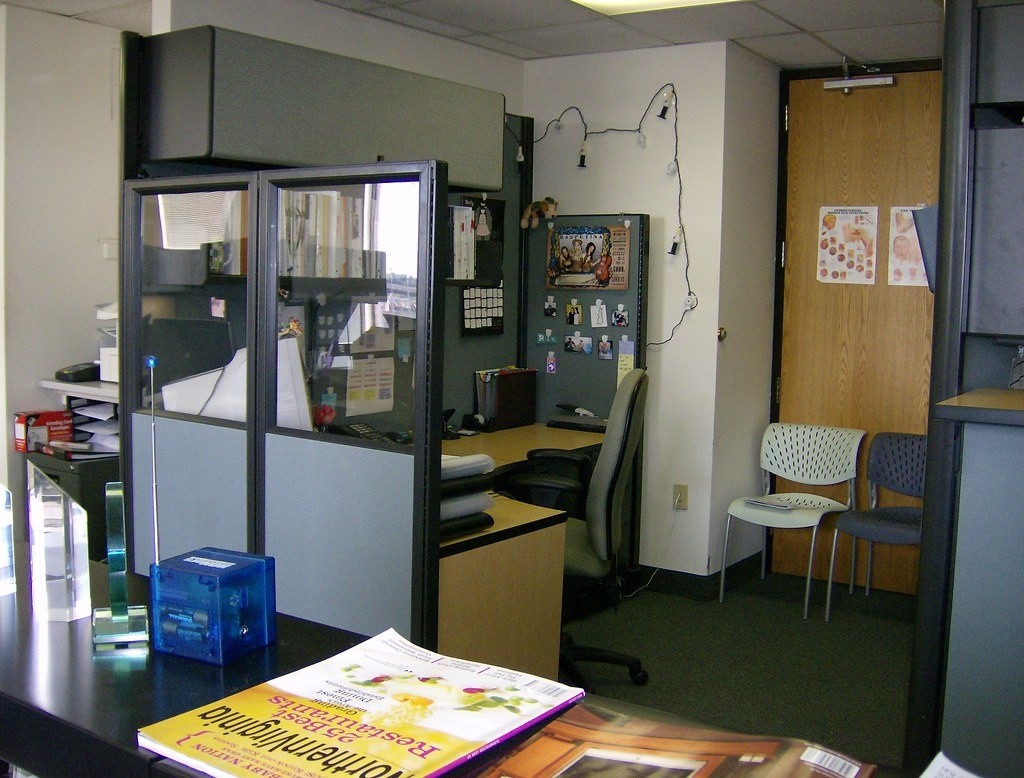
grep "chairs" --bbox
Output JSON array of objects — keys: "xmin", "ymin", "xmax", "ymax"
[
  {"xmin": 512, "ymin": 369, "xmax": 649, "ymax": 687},
  {"xmin": 718, "ymin": 422, "xmax": 866, "ymax": 620},
  {"xmin": 825, "ymin": 432, "xmax": 928, "ymax": 623}
]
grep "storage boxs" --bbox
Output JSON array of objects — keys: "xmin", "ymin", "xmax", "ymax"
[{"xmin": 15, "ymin": 411, "xmax": 75, "ymax": 452}]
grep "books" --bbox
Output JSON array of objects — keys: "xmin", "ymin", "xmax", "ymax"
[
  {"xmin": 135, "ymin": 627, "xmax": 983, "ymax": 778},
  {"xmin": 34, "ymin": 440, "xmax": 121, "ymax": 462}
]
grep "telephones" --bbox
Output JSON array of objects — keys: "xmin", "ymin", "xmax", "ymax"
[{"xmin": 327, "ymin": 422, "xmax": 396, "ymax": 445}]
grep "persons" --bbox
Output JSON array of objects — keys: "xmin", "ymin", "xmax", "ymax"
[{"xmin": 542, "ymin": 301, "xmax": 628, "ymax": 378}]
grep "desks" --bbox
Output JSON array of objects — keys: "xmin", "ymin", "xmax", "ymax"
[{"xmin": 410, "ymin": 423, "xmax": 605, "ymax": 683}]
